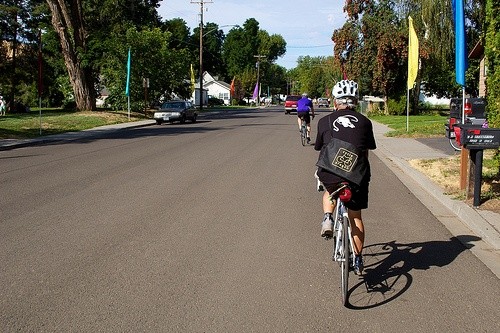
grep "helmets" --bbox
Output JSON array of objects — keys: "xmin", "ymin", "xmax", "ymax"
[
  {"xmin": 332, "ymin": 79, "xmax": 359, "ymax": 98},
  {"xmin": 301, "ymin": 93, "xmax": 308, "ymax": 98}
]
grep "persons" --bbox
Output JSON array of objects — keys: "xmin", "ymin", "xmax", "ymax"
[
  {"xmin": 296, "ymin": 93, "xmax": 315, "ymax": 143},
  {"xmin": 0, "ymin": 96, "xmax": 7, "ymax": 116},
  {"xmin": 314, "ymin": 80, "xmax": 377, "ymax": 274}
]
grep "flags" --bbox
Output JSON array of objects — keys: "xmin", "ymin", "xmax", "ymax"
[
  {"xmin": 125, "ymin": 48, "xmax": 131, "ymax": 97},
  {"xmin": 252, "ymin": 83, "xmax": 258, "ymax": 99},
  {"xmin": 452, "ymin": 0, "xmax": 469, "ymax": 87},
  {"xmin": 407, "ymin": 15, "xmax": 419, "ymax": 89},
  {"xmin": 231, "ymin": 78, "xmax": 236, "ymax": 96},
  {"xmin": 189, "ymin": 65, "xmax": 195, "ymax": 94}
]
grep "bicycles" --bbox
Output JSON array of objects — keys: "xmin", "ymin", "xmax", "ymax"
[
  {"xmin": 323, "ymin": 181, "xmax": 364, "ymax": 305},
  {"xmin": 299, "ymin": 114, "xmax": 315, "ymax": 146}
]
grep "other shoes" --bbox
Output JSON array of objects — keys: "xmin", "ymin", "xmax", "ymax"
[
  {"xmin": 299, "ymin": 129, "xmax": 302, "ymax": 132},
  {"xmin": 353, "ymin": 256, "xmax": 364, "ymax": 276},
  {"xmin": 307, "ymin": 136, "xmax": 310, "ymax": 141},
  {"xmin": 320, "ymin": 214, "xmax": 335, "ymax": 238}
]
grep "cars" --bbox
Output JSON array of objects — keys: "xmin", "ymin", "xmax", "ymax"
[
  {"xmin": 208, "ymin": 95, "xmax": 224, "ymax": 108},
  {"xmin": 154, "ymin": 101, "xmax": 199, "ymax": 125}
]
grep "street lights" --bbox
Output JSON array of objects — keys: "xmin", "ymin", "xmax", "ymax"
[{"xmin": 199, "ymin": 24, "xmax": 240, "ymax": 109}]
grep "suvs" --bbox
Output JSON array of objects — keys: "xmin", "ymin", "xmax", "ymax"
[{"xmin": 284, "ymin": 94, "xmax": 302, "ymax": 115}]
